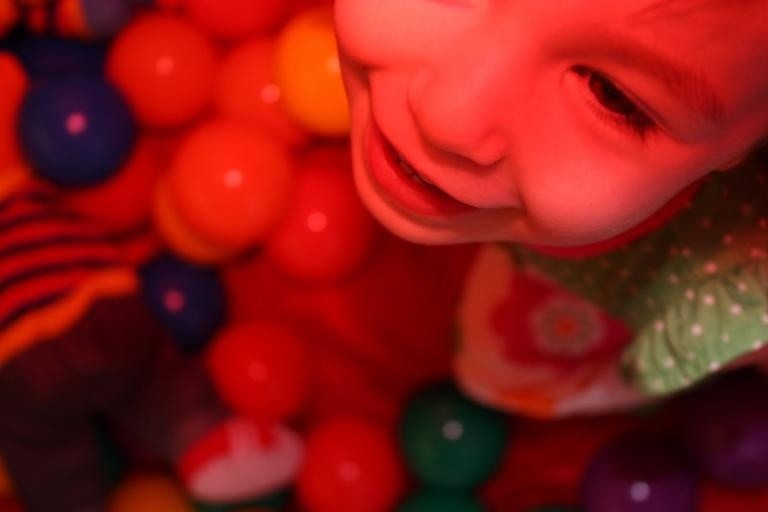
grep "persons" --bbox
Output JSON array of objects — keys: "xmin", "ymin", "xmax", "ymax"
[
  {"xmin": 0, "ymin": 55, "xmax": 308, "ymax": 507},
  {"xmin": 332, "ymin": 0, "xmax": 768, "ymax": 419}
]
